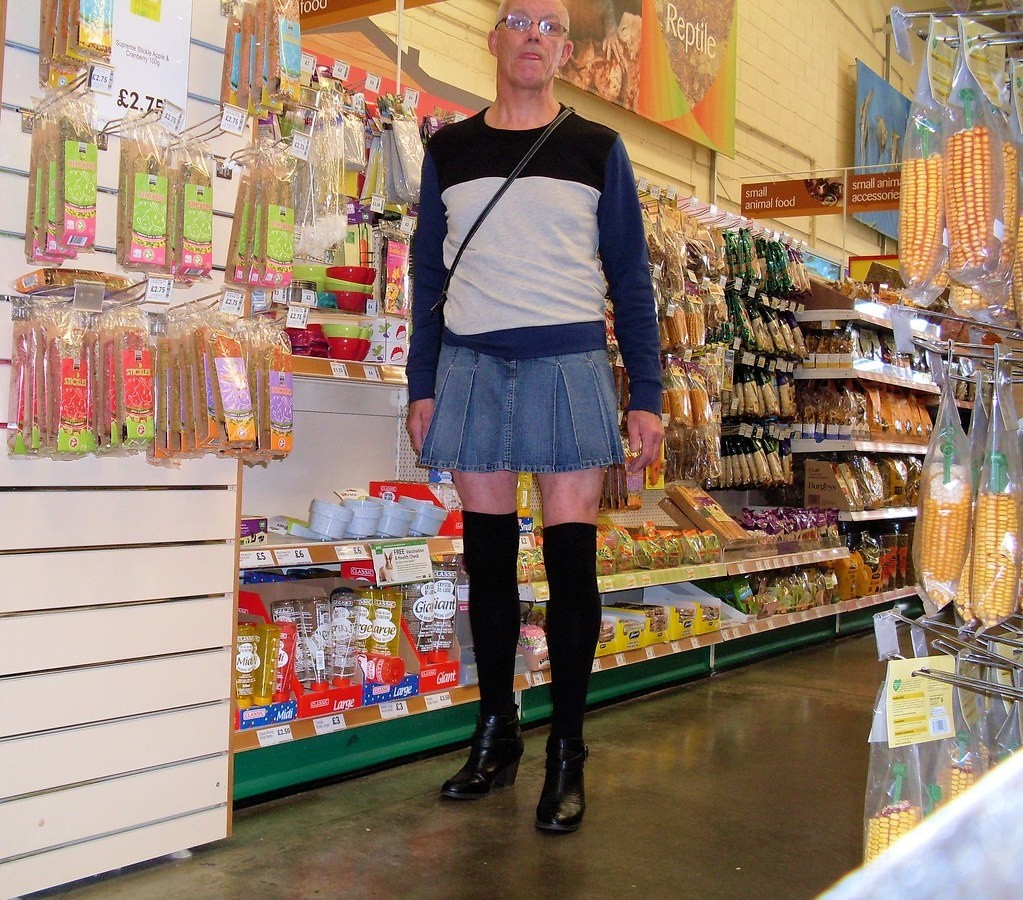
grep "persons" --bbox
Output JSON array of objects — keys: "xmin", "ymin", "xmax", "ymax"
[{"xmin": 402, "ymin": 1, "xmax": 668, "ymax": 836}]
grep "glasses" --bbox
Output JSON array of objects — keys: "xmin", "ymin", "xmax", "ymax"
[{"xmin": 494, "ymin": 13, "xmax": 568, "ymax": 39}]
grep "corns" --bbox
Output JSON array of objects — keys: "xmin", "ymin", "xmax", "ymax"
[
  {"xmin": 897, "ymin": 125, "xmax": 1023, "ymax": 332},
  {"xmin": 656, "ymin": 310, "xmax": 710, "ymax": 429},
  {"xmin": 863, "ymin": 739, "xmax": 989, "ymax": 866},
  {"xmin": 910, "ymin": 464, "xmax": 1023, "ymax": 632}
]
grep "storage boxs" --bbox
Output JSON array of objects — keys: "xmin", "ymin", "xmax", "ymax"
[{"xmin": 234, "ymin": 261, "xmax": 941, "ymax": 732}]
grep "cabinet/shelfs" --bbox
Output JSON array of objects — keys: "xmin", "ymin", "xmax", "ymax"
[{"xmin": 235, "ymin": 308, "xmax": 973, "ymax": 754}]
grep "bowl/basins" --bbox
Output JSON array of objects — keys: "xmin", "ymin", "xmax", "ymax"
[
  {"xmin": 365, "ymin": 496, "xmax": 417, "ymax": 538},
  {"xmin": 398, "ymin": 496, "xmax": 449, "ymax": 536},
  {"xmin": 282, "ymin": 264, "xmax": 377, "ymax": 361},
  {"xmin": 340, "ymin": 498, "xmax": 384, "ymax": 535},
  {"xmin": 308, "ymin": 498, "xmax": 355, "ymax": 539}
]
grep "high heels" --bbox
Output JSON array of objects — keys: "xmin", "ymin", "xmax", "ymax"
[
  {"xmin": 441, "ymin": 703, "xmax": 525, "ymax": 799},
  {"xmin": 536, "ymin": 735, "xmax": 589, "ymax": 831}
]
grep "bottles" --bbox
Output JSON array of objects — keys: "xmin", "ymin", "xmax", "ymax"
[
  {"xmin": 432, "ymin": 561, "xmax": 461, "ymax": 651},
  {"xmin": 401, "ymin": 580, "xmax": 432, "ymax": 653},
  {"xmin": 234, "ymin": 586, "xmax": 405, "ymax": 709}
]
